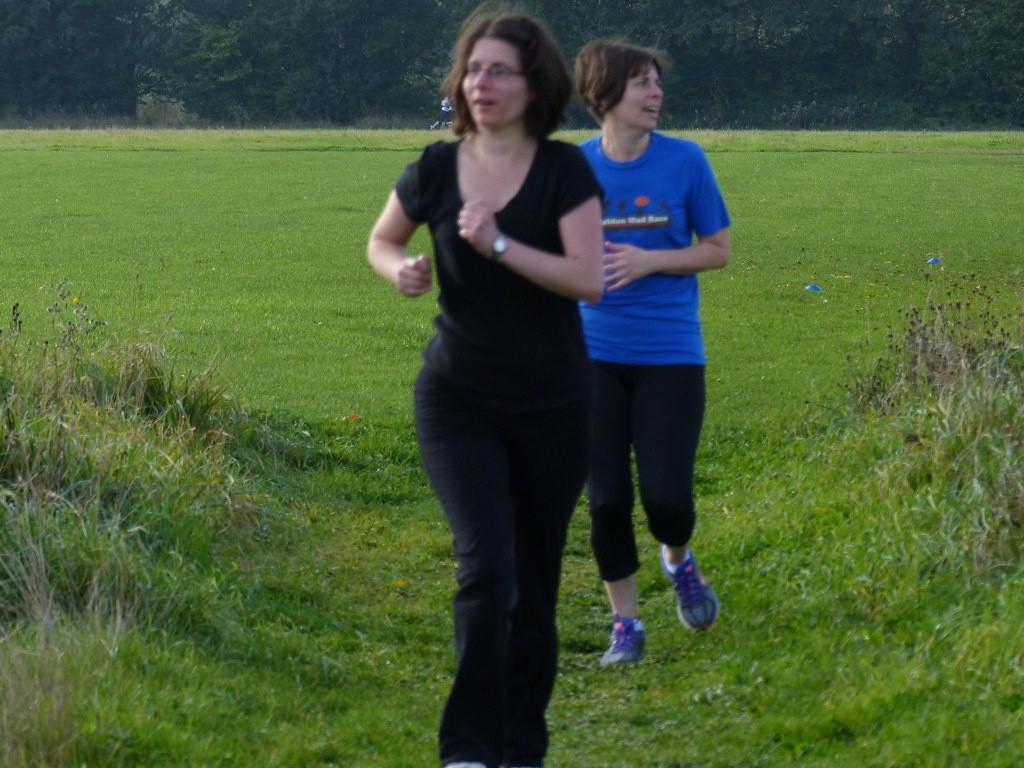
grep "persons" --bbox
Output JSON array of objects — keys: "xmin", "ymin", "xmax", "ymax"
[
  {"xmin": 565, "ymin": 36, "xmax": 731, "ymax": 670},
  {"xmin": 360, "ymin": 11, "xmax": 607, "ymax": 768}
]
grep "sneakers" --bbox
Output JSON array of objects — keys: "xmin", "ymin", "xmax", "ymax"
[
  {"xmin": 663, "ymin": 542, "xmax": 717, "ymax": 629},
  {"xmin": 603, "ymin": 612, "xmax": 646, "ymax": 667}
]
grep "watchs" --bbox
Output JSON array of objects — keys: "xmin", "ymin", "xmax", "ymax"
[{"xmin": 490, "ymin": 233, "xmax": 510, "ymax": 261}]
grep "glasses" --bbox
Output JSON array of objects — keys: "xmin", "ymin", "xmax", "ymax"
[{"xmin": 465, "ymin": 64, "xmax": 525, "ymax": 80}]
